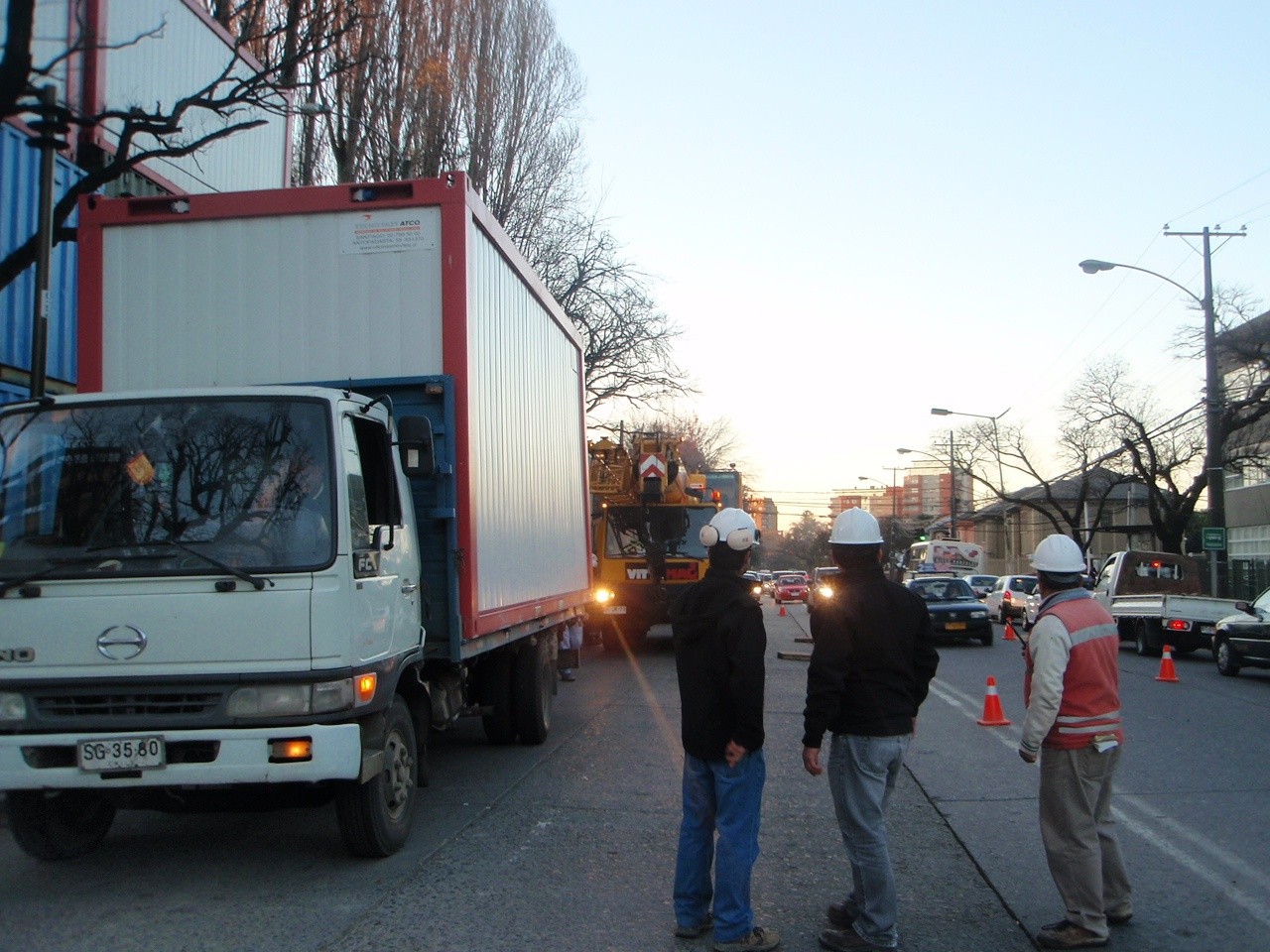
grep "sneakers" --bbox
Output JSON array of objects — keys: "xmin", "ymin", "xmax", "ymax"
[
  {"xmin": 1038, "ymin": 919, "xmax": 1110, "ymax": 947},
  {"xmin": 827, "ymin": 903, "xmax": 856, "ymax": 927},
  {"xmin": 561, "ymin": 673, "xmax": 575, "ymax": 681},
  {"xmin": 818, "ymin": 925, "xmax": 898, "ymax": 952},
  {"xmin": 674, "ymin": 911, "xmax": 715, "ymax": 938},
  {"xmin": 1102, "ymin": 911, "xmax": 1134, "ymax": 922},
  {"xmin": 713, "ymin": 925, "xmax": 780, "ymax": 952}
]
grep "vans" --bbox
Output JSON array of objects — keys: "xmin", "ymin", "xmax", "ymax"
[{"xmin": 899, "ymin": 540, "xmax": 984, "ymax": 596}]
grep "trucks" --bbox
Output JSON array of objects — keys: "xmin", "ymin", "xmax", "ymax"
[
  {"xmin": 0, "ymin": 169, "xmax": 602, "ymax": 863},
  {"xmin": 1087, "ymin": 548, "xmax": 1252, "ymax": 657}
]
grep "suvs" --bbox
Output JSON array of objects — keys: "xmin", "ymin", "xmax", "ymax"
[{"xmin": 806, "ymin": 566, "xmax": 842, "ymax": 613}]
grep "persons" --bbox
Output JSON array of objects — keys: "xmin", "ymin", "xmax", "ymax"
[
  {"xmin": 1020, "ymin": 533, "xmax": 1135, "ymax": 947},
  {"xmin": 672, "ymin": 506, "xmax": 780, "ymax": 952},
  {"xmin": 275, "ymin": 452, "xmax": 330, "ymax": 545},
  {"xmin": 946, "ymin": 585, "xmax": 962, "ymax": 597},
  {"xmin": 802, "ymin": 506, "xmax": 938, "ymax": 952},
  {"xmin": 556, "ymin": 554, "xmax": 597, "ymax": 684},
  {"xmin": 917, "ymin": 587, "xmax": 937, "ymax": 599}
]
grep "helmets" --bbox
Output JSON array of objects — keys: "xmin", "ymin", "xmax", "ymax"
[
  {"xmin": 827, "ymin": 506, "xmax": 885, "ymax": 545},
  {"xmin": 1030, "ymin": 534, "xmax": 1087, "ymax": 573},
  {"xmin": 707, "ymin": 506, "xmax": 760, "ymax": 545}
]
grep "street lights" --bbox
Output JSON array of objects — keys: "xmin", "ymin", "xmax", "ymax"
[
  {"xmin": 896, "ymin": 448, "xmax": 957, "ymax": 541},
  {"xmin": 930, "ymin": 408, "xmax": 1014, "ymax": 576},
  {"xmin": 1078, "ymin": 257, "xmax": 1229, "ymax": 598},
  {"xmin": 858, "ymin": 476, "xmax": 897, "ymax": 582}
]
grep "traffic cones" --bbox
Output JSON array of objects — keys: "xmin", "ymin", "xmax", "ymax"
[
  {"xmin": 1153, "ymin": 644, "xmax": 1180, "ymax": 683},
  {"xmin": 1000, "ymin": 617, "xmax": 1018, "ymax": 640},
  {"xmin": 777, "ymin": 603, "xmax": 787, "ymax": 616},
  {"xmin": 976, "ymin": 676, "xmax": 1012, "ymax": 726}
]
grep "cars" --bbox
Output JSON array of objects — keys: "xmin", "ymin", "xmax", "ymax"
[
  {"xmin": 901, "ymin": 575, "xmax": 993, "ymax": 647},
  {"xmin": 1020, "ymin": 574, "xmax": 1099, "ymax": 631},
  {"xmin": 961, "ymin": 574, "xmax": 1001, "ymax": 596},
  {"xmin": 980, "ymin": 575, "xmax": 1040, "ymax": 625},
  {"xmin": 1211, "ymin": 585, "xmax": 1270, "ymax": 677},
  {"xmin": 741, "ymin": 568, "xmax": 811, "ymax": 605}
]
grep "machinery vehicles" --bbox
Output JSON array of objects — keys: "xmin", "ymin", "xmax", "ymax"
[{"xmin": 586, "ymin": 421, "xmax": 745, "ymax": 652}]
